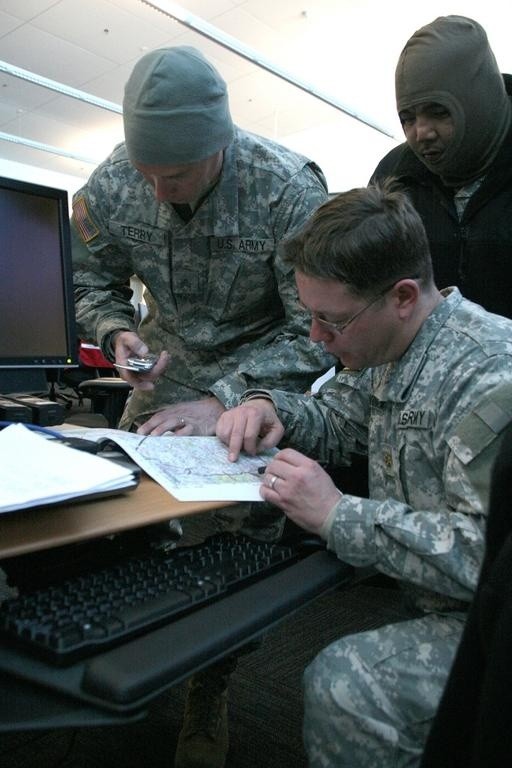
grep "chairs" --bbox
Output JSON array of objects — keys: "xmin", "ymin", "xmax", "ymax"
[{"xmin": 410, "ymin": 427, "xmax": 511, "ymax": 764}]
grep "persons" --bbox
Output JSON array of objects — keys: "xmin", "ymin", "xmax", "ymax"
[
  {"xmin": 208, "ymin": 185, "xmax": 511, "ymax": 766},
  {"xmin": 361, "ymin": 14, "xmax": 512, "ymax": 324},
  {"xmin": 64, "ymin": 44, "xmax": 343, "ymax": 767}
]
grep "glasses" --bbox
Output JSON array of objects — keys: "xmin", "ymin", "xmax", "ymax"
[{"xmin": 295, "ymin": 275, "xmax": 420, "ymax": 335}]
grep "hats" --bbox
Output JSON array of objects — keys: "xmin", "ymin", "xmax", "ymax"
[
  {"xmin": 123, "ymin": 47, "xmax": 232, "ymax": 165},
  {"xmin": 395, "ymin": 16, "xmax": 512, "ymax": 187}
]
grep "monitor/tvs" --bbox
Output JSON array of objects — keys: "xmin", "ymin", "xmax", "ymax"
[{"xmin": 1, "ymin": 176, "xmax": 80, "ymax": 423}]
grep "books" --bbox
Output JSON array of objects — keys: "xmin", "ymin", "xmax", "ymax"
[{"xmin": 39, "ymin": 421, "xmax": 287, "ymax": 503}]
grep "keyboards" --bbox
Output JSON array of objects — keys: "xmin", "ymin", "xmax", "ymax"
[{"xmin": 0, "ymin": 531, "xmax": 350, "ymax": 716}]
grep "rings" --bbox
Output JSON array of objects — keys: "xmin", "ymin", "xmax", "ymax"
[
  {"xmin": 177, "ymin": 414, "xmax": 184, "ymax": 426},
  {"xmin": 266, "ymin": 473, "xmax": 278, "ymax": 489}
]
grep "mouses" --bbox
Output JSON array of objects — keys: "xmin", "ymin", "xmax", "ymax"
[{"xmin": 282, "ymin": 531, "xmax": 326, "ymax": 556}]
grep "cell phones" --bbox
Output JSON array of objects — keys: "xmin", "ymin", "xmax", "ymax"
[{"xmin": 112, "ymin": 352, "xmax": 159, "ymax": 374}]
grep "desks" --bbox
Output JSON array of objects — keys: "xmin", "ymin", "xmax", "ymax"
[{"xmin": 0, "ymin": 430, "xmax": 342, "ymax": 558}]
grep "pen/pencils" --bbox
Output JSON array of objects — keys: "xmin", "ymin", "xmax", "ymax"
[{"xmin": 250, "ymin": 459, "xmax": 330, "ymax": 475}]
